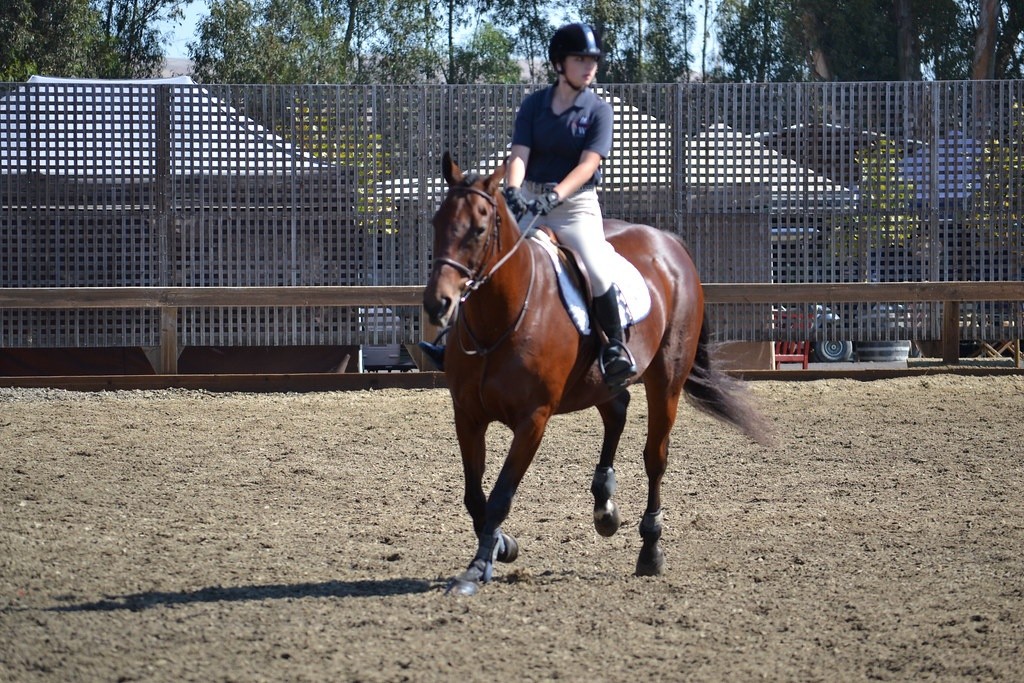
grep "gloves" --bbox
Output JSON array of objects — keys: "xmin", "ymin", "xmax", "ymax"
[{"xmin": 506, "ymin": 187, "xmax": 562, "ymax": 217}]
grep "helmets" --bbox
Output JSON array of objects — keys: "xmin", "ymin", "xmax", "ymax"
[{"xmin": 549, "ymin": 23, "xmax": 602, "ymax": 62}]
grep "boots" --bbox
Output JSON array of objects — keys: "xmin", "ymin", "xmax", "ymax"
[
  {"xmin": 591, "ymin": 285, "xmax": 631, "ymax": 390},
  {"xmin": 418, "ymin": 341, "xmax": 445, "ymax": 372}
]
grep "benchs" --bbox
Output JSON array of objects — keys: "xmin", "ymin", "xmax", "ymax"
[{"xmin": 772, "ymin": 311, "xmax": 814, "ymax": 369}]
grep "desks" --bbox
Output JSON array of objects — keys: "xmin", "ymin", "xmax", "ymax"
[{"xmin": 957, "ymin": 316, "xmax": 1024, "ymax": 361}]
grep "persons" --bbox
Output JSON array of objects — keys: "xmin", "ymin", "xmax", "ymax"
[{"xmin": 504, "ymin": 24, "xmax": 633, "ymax": 385}]
